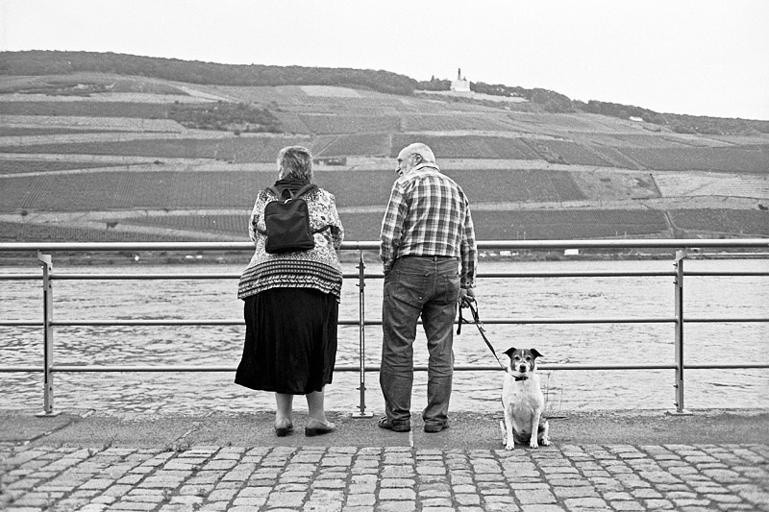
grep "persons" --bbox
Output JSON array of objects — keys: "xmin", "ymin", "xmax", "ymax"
[
  {"xmin": 234, "ymin": 145, "xmax": 346, "ymax": 437},
  {"xmin": 378, "ymin": 141, "xmax": 479, "ymax": 434}
]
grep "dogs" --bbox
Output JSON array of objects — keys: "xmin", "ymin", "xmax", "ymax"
[{"xmin": 499, "ymin": 346, "xmax": 551, "ymax": 449}]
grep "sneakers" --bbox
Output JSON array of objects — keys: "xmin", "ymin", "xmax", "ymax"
[
  {"xmin": 273, "ymin": 413, "xmax": 295, "ymax": 437},
  {"xmin": 304, "ymin": 418, "xmax": 336, "ymax": 436}
]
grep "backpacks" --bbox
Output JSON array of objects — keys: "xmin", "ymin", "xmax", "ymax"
[{"xmin": 264, "ymin": 185, "xmax": 317, "ymax": 253}]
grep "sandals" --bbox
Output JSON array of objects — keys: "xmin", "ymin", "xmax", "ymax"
[
  {"xmin": 376, "ymin": 415, "xmax": 411, "ymax": 432},
  {"xmin": 424, "ymin": 415, "xmax": 451, "ymax": 434}
]
